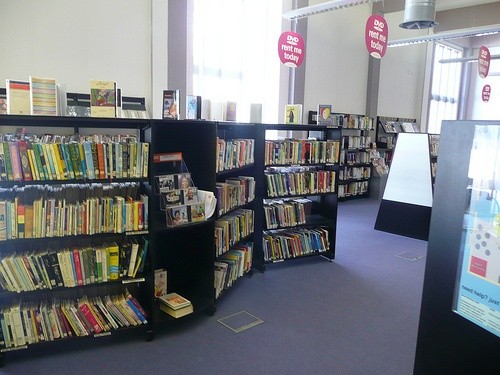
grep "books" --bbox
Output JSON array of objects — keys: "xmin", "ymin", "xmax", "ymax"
[
  {"xmin": 159, "ymin": 172, "xmax": 218, "ymax": 228},
  {"xmin": 216, "ymin": 136, "xmax": 255, "ymax": 174},
  {"xmin": 262, "ymin": 228, "xmax": 330, "ymax": 263},
  {"xmin": 214, "ymin": 209, "xmax": 255, "ymax": 258},
  {"xmin": 285, "ymin": 104, "xmax": 302, "ymax": 124},
  {"xmin": 317, "ymin": 103, "xmax": 441, "ymax": 198},
  {"xmin": 264, "ymin": 137, "xmax": 340, "ymax": 167},
  {"xmin": 154, "ymin": 268, "xmax": 168, "ymax": 298},
  {"xmin": 216, "ymin": 176, "xmax": 256, "ymax": 218},
  {"xmin": 0, "ymin": 128, "xmax": 150, "ymax": 181},
  {"xmin": 0, "ymin": 194, "xmax": 148, "ymax": 241},
  {"xmin": 213, "ymin": 242, "xmax": 254, "ymax": 299},
  {"xmin": 265, "ymin": 170, "xmax": 336, "ymax": 197},
  {"xmin": 263, "ymin": 198, "xmax": 312, "ymax": 230},
  {"xmin": 159, "ymin": 292, "xmax": 194, "ymax": 320},
  {"xmin": 0, "ymin": 287, "xmax": 152, "ymax": 352},
  {"xmin": 90, "ymin": 80, "xmax": 118, "ymax": 118},
  {"xmin": 0, "ymin": 236, "xmax": 150, "ymax": 294},
  {"xmin": 6, "ymin": 76, "xmax": 67, "ymax": 116},
  {"xmin": 160, "ymin": 90, "xmax": 265, "ymax": 125}
]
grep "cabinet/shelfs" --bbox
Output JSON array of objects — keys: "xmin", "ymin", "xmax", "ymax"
[
  {"xmin": 307, "ymin": 111, "xmax": 441, "ymax": 242},
  {"xmin": 0, "ymin": 115, "xmax": 344, "ymax": 365}
]
